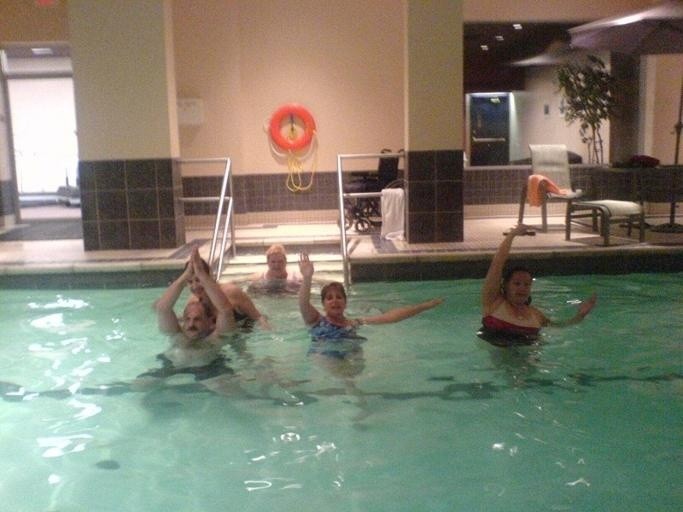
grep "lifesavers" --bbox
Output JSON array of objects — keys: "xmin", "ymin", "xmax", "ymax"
[{"xmin": 269, "ymin": 104, "xmax": 316, "ymax": 150}]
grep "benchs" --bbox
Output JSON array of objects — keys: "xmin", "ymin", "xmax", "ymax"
[{"xmin": 564, "ymin": 197, "xmax": 646, "ymax": 248}]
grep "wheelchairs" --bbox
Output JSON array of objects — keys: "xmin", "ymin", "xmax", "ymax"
[{"xmin": 338, "ymin": 148, "xmax": 401, "ymax": 231}]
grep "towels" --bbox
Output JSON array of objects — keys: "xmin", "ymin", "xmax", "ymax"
[
  {"xmin": 527, "ymin": 172, "xmax": 566, "ymax": 206},
  {"xmin": 379, "ymin": 188, "xmax": 405, "ymax": 241}
]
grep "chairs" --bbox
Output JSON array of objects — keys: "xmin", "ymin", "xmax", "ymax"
[{"xmin": 518, "ymin": 142, "xmax": 598, "ymax": 234}]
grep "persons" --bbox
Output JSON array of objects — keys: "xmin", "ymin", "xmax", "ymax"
[
  {"xmin": 297, "ymin": 251, "xmax": 443, "ymax": 379},
  {"xmin": 245, "ymin": 244, "xmax": 304, "ymax": 294},
  {"xmin": 475, "ymin": 223, "xmax": 597, "ymax": 349},
  {"xmin": 183, "ymin": 259, "xmax": 272, "ymax": 331},
  {"xmin": 130, "ymin": 246, "xmax": 245, "ymax": 397}
]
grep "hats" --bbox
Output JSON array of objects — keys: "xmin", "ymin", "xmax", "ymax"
[{"xmin": 266, "ymin": 244, "xmax": 285, "ymax": 260}]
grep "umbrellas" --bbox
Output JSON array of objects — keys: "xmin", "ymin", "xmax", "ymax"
[{"xmin": 567, "ymin": 3, "xmax": 683, "ymax": 232}]
